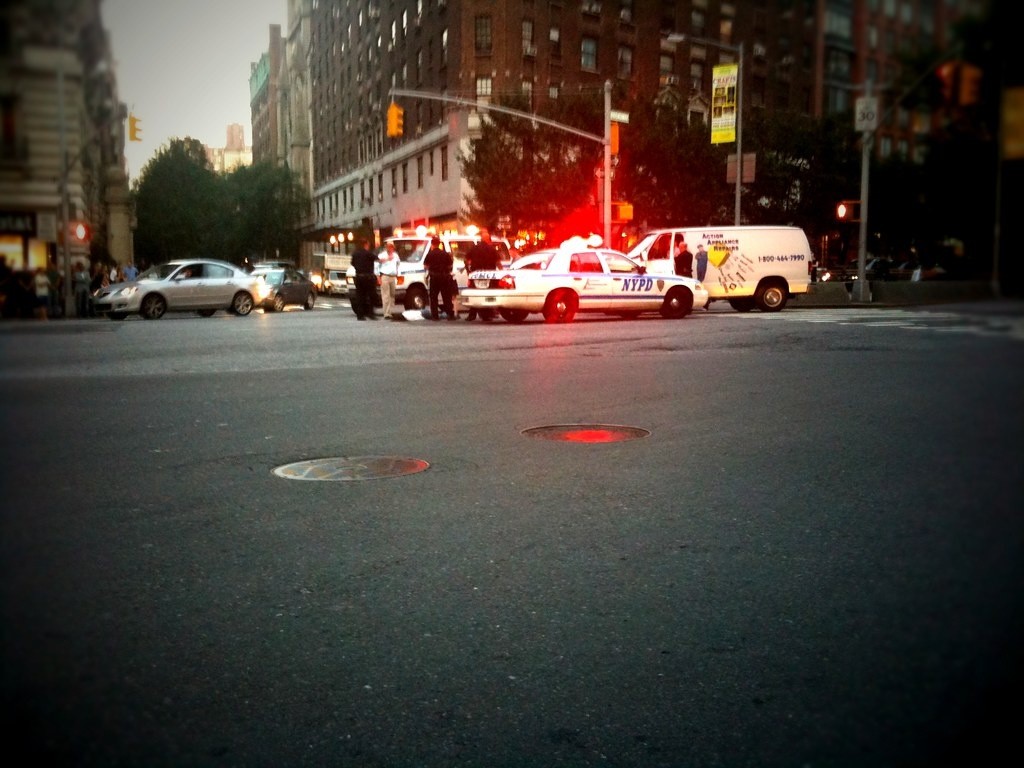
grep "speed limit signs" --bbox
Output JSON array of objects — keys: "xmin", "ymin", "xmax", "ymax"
[{"xmin": 855, "ymin": 98, "xmax": 877, "ymax": 131}]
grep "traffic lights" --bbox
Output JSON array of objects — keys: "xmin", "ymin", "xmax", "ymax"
[
  {"xmin": 71, "ymin": 223, "xmax": 89, "ymax": 241},
  {"xmin": 387, "ymin": 104, "xmax": 403, "ymax": 137},
  {"xmin": 128, "ymin": 116, "xmax": 142, "ymax": 141},
  {"xmin": 836, "ymin": 203, "xmax": 847, "ymax": 220}
]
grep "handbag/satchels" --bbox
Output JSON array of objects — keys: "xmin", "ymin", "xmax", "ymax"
[{"xmin": 451, "ymin": 280, "xmax": 459, "ymax": 295}]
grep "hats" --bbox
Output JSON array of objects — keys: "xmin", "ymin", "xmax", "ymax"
[
  {"xmin": 387, "ymin": 241, "xmax": 396, "ymax": 248},
  {"xmin": 431, "ymin": 237, "xmax": 440, "ymax": 244}
]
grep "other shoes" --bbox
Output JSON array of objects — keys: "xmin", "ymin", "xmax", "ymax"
[
  {"xmin": 385, "ymin": 316, "xmax": 391, "ymax": 320},
  {"xmin": 482, "ymin": 317, "xmax": 493, "ymax": 321},
  {"xmin": 357, "ymin": 317, "xmax": 366, "ymax": 321},
  {"xmin": 465, "ymin": 316, "xmax": 475, "ymax": 321},
  {"xmin": 370, "ymin": 317, "xmax": 379, "ymax": 321},
  {"xmin": 431, "ymin": 316, "xmax": 440, "ymax": 321},
  {"xmin": 446, "ymin": 316, "xmax": 457, "ymax": 321}
]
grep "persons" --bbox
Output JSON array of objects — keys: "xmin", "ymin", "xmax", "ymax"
[
  {"xmin": 0, "ymin": 255, "xmax": 139, "ymax": 321},
  {"xmin": 424, "ymin": 227, "xmax": 508, "ymax": 322},
  {"xmin": 352, "ymin": 237, "xmax": 393, "ymax": 322},
  {"xmin": 665, "ymin": 239, "xmax": 693, "ymax": 277},
  {"xmin": 184, "ymin": 266, "xmax": 192, "ymax": 278},
  {"xmin": 378, "ymin": 241, "xmax": 401, "ymax": 319}
]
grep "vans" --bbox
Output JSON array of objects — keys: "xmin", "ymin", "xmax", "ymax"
[
  {"xmin": 624, "ymin": 225, "xmax": 813, "ymax": 312},
  {"xmin": 347, "ymin": 235, "xmax": 515, "ymax": 315}
]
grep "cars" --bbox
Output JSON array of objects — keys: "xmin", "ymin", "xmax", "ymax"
[
  {"xmin": 321, "ymin": 269, "xmax": 348, "ymax": 299},
  {"xmin": 94, "ymin": 259, "xmax": 265, "ymax": 321},
  {"xmin": 846, "ymin": 259, "xmax": 882, "ymax": 290},
  {"xmin": 461, "ymin": 247, "xmax": 706, "ymax": 323},
  {"xmin": 250, "ymin": 269, "xmax": 317, "ymax": 313}
]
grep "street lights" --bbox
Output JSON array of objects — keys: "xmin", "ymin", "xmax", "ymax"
[{"xmin": 668, "ymin": 33, "xmax": 743, "ymax": 226}]
